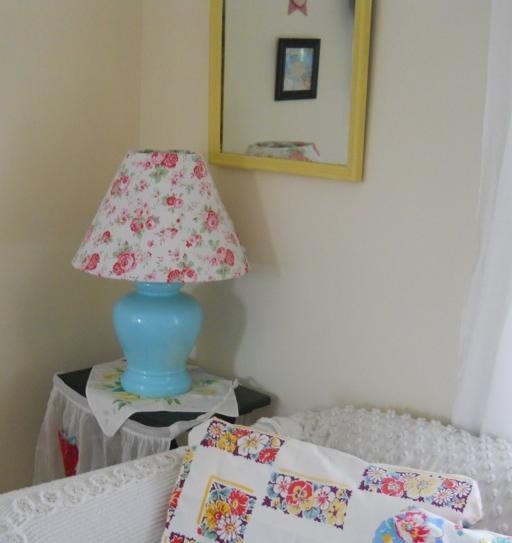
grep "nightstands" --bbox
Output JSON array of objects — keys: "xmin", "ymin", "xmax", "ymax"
[{"xmin": 42, "ymin": 356, "xmax": 274, "ymax": 479}]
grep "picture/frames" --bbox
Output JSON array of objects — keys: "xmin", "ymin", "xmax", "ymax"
[{"xmin": 272, "ymin": 35, "xmax": 322, "ymax": 100}]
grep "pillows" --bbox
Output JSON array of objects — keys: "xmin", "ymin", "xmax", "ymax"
[
  {"xmin": 159, "ymin": 414, "xmax": 489, "ymax": 542},
  {"xmin": 375, "ymin": 508, "xmax": 512, "ymax": 543}
]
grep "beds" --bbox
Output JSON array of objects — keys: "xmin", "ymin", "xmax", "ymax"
[{"xmin": 1, "ymin": 402, "xmax": 511, "ymax": 542}]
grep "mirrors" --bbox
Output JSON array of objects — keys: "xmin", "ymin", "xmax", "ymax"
[{"xmin": 206, "ymin": 0, "xmax": 376, "ymax": 184}]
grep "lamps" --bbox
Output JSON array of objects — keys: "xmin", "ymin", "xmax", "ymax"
[
  {"xmin": 68, "ymin": 146, "xmax": 252, "ymax": 398},
  {"xmin": 243, "ymin": 139, "xmax": 322, "ymax": 165}
]
